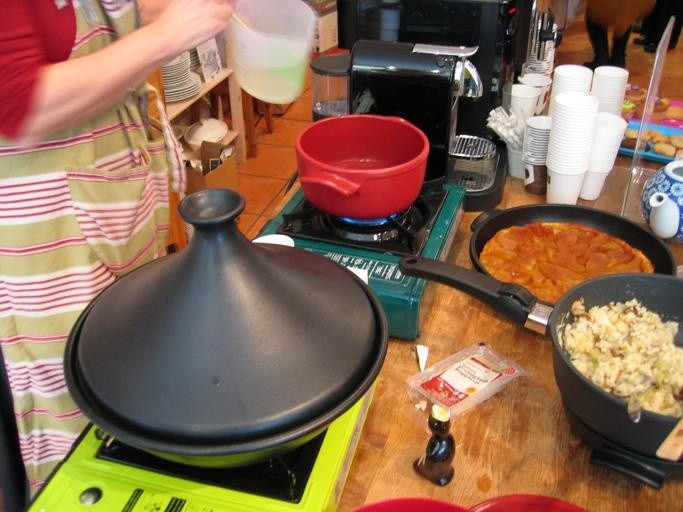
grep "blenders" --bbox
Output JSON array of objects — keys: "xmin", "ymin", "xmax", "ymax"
[{"xmin": 312, "ymin": 40, "xmax": 503, "ymax": 211}]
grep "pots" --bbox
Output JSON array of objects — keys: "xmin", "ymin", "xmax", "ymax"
[
  {"xmin": 399, "ymin": 257, "xmax": 682, "ymax": 491},
  {"xmin": 62, "ymin": 188, "xmax": 390, "ymax": 469},
  {"xmin": 295, "ymin": 114, "xmax": 430, "ymax": 219},
  {"xmin": 469, "ymin": 204, "xmax": 677, "ymax": 307}
]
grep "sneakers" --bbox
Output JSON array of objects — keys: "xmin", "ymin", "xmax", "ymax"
[
  {"xmin": 634, "ymin": 38, "xmax": 649, "ymax": 45},
  {"xmin": 645, "ymin": 40, "xmax": 678, "ymax": 52}
]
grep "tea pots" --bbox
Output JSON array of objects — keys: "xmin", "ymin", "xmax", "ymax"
[{"xmin": 640, "ymin": 159, "xmax": 683, "ymax": 238}]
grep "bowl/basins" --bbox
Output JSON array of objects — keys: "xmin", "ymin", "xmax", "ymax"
[{"xmin": 183, "ymin": 119, "xmax": 228, "ymax": 152}]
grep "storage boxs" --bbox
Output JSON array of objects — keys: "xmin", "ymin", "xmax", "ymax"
[{"xmin": 183, "ymin": 141, "xmax": 238, "ymax": 201}]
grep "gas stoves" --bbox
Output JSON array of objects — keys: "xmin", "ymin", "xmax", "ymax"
[{"xmin": 249, "ymin": 175, "xmax": 468, "ymax": 344}]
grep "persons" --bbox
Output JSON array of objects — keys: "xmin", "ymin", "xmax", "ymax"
[
  {"xmin": 632, "ymin": 0, "xmax": 683, "ymax": 54},
  {"xmin": 1, "ymin": 0, "xmax": 235, "ymax": 511},
  {"xmin": 579, "ymin": 0, "xmax": 660, "ymax": 66}
]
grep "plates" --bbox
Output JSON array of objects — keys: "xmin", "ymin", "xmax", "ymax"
[
  {"xmin": 526, "ymin": 20, "xmax": 558, "ymax": 75},
  {"xmin": 617, "ymin": 120, "xmax": 683, "ymax": 163},
  {"xmin": 160, "ymin": 51, "xmax": 201, "ymax": 103}
]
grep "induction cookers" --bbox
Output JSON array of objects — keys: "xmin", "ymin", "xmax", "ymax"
[{"xmin": 23, "ymin": 366, "xmax": 380, "ymax": 511}]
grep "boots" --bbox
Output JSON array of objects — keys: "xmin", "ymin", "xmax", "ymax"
[
  {"xmin": 611, "ymin": 25, "xmax": 631, "ymax": 68},
  {"xmin": 583, "ymin": 13, "xmax": 610, "ymax": 71}
]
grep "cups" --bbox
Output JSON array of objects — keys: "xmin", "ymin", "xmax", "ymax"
[
  {"xmin": 224, "ymin": 0, "xmax": 315, "ymax": 105},
  {"xmin": 505, "ymin": 64, "xmax": 629, "ymax": 206}
]
